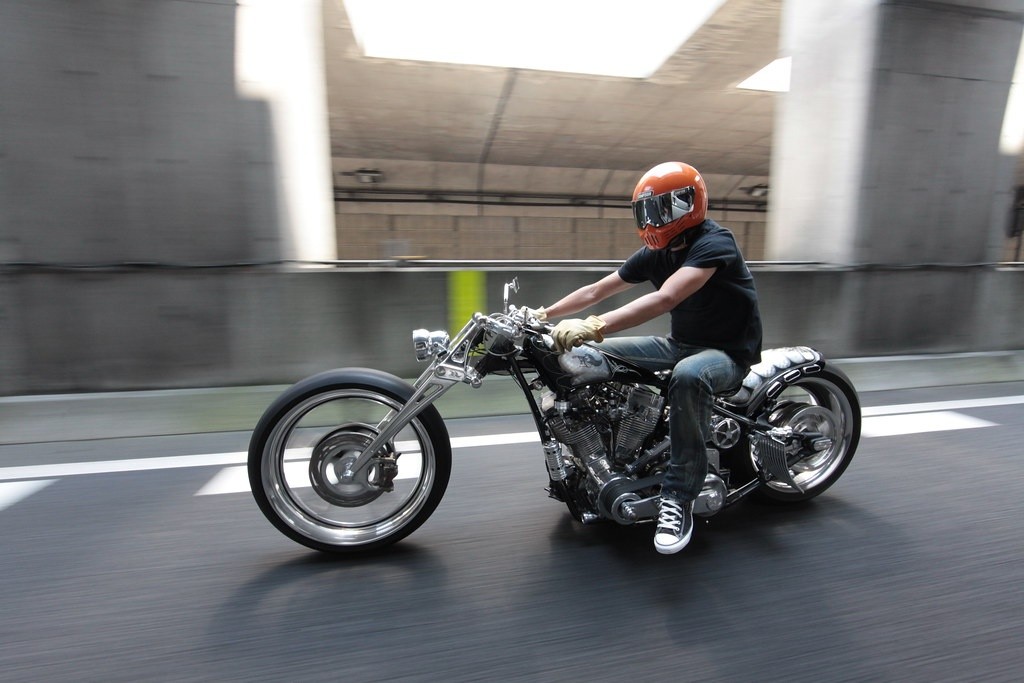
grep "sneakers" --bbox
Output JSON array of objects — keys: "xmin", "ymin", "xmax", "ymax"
[{"xmin": 654, "ymin": 494, "xmax": 697, "ymax": 555}]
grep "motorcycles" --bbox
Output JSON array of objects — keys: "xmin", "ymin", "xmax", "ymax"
[{"xmin": 247, "ymin": 274, "xmax": 862, "ymax": 558}]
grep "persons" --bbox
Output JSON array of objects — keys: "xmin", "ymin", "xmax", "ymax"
[{"xmin": 518, "ymin": 162, "xmax": 764, "ymax": 555}]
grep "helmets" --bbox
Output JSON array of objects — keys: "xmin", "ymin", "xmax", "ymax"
[{"xmin": 631, "ymin": 160, "xmax": 707, "ymax": 251}]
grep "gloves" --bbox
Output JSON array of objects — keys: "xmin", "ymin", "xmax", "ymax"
[
  {"xmin": 522, "ymin": 304, "xmax": 548, "ymax": 325},
  {"xmin": 553, "ymin": 315, "xmax": 607, "ymax": 354}
]
